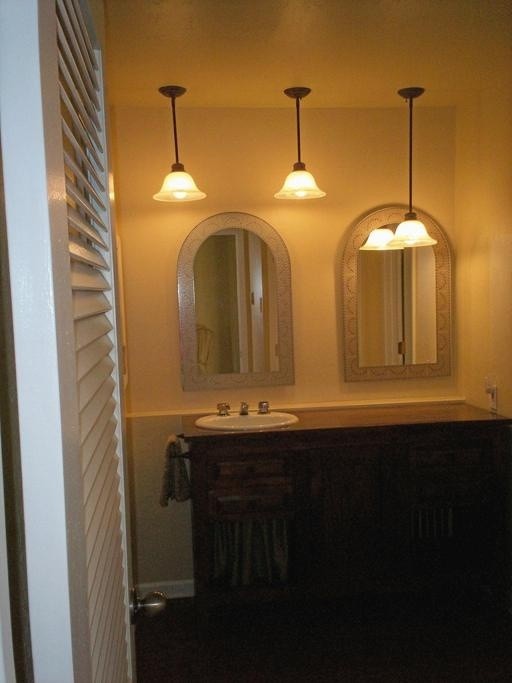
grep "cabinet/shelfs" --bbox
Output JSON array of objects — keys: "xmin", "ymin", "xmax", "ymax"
[{"xmin": 180, "ymin": 427, "xmax": 510, "ymax": 641}]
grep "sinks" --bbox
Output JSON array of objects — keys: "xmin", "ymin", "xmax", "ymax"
[{"xmin": 194, "ymin": 410, "xmax": 299, "ymax": 431}]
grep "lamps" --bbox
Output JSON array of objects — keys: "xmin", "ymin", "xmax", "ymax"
[
  {"xmin": 359, "ymin": 227, "xmax": 405, "ymax": 253},
  {"xmin": 152, "ymin": 86, "xmax": 207, "ymax": 204},
  {"xmin": 387, "ymin": 86, "xmax": 439, "ymax": 249},
  {"xmin": 272, "ymin": 86, "xmax": 328, "ymax": 200}
]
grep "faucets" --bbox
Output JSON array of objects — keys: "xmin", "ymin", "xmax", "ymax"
[{"xmin": 240, "ymin": 400, "xmax": 249, "ymax": 414}]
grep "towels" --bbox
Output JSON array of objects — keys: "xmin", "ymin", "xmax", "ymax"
[{"xmin": 156, "ymin": 434, "xmax": 193, "ymax": 509}]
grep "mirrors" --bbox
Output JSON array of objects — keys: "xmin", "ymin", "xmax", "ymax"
[
  {"xmin": 175, "ymin": 210, "xmax": 299, "ymax": 391},
  {"xmin": 332, "ymin": 204, "xmax": 458, "ymax": 386}
]
